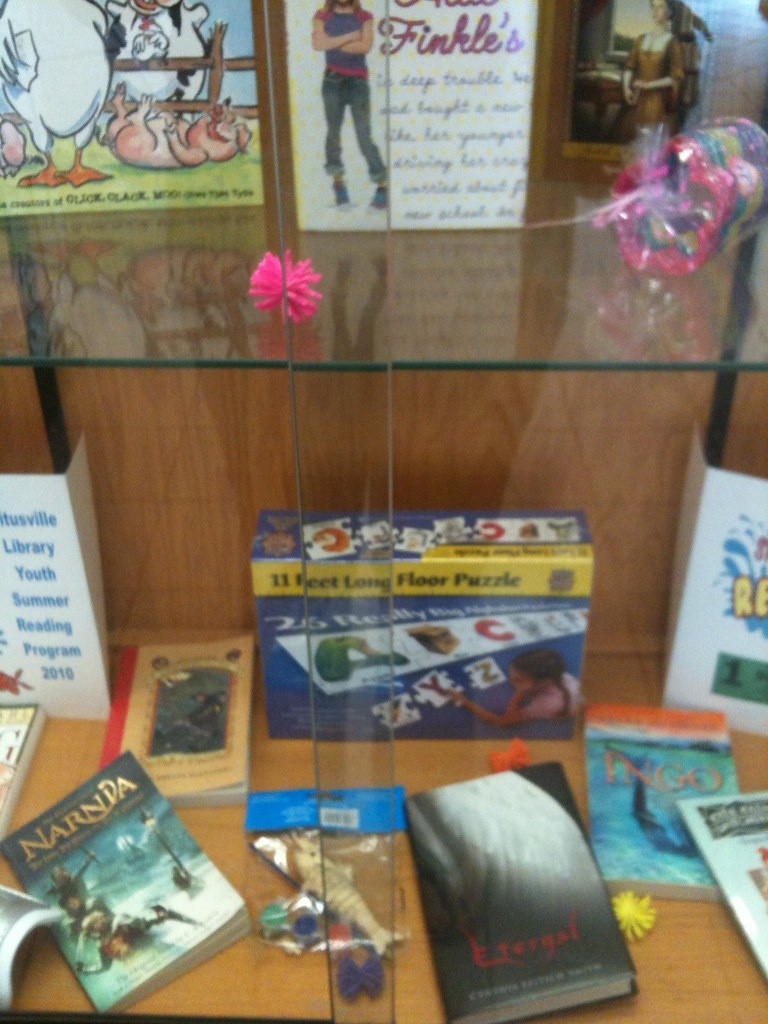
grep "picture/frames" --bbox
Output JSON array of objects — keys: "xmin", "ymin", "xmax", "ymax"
[{"xmin": 554, "ymin": 0, "xmax": 724, "ymax": 169}]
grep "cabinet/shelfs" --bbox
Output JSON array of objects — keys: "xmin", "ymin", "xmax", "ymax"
[{"xmin": 0, "ymin": 0, "xmax": 767, "ymax": 1024}]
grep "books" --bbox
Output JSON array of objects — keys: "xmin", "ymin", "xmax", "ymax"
[
  {"xmin": 407, "ymin": 759, "xmax": 640, "ymax": 1021},
  {"xmin": 2, "ymin": 749, "xmax": 254, "ymax": 1014},
  {"xmin": 678, "ymin": 789, "xmax": 768, "ymax": 985},
  {"xmin": 98, "ymin": 634, "xmax": 252, "ymax": 814},
  {"xmin": 581, "ymin": 699, "xmax": 746, "ymax": 904}
]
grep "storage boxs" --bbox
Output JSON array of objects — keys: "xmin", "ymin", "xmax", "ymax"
[{"xmin": 249, "ymin": 511, "xmax": 594, "ymax": 739}]
